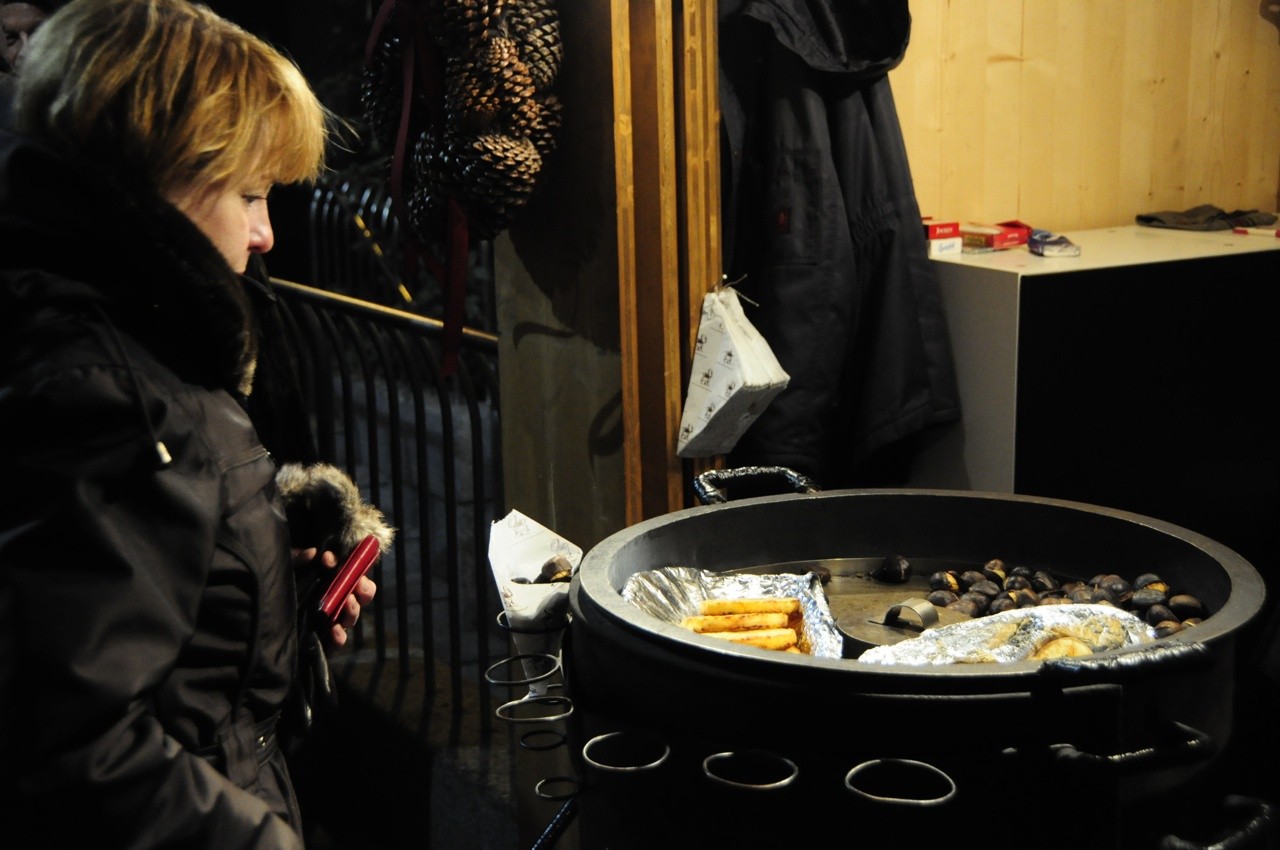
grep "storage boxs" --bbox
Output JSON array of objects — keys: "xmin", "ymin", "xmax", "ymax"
[{"xmin": 922, "ymin": 216, "xmax": 1032, "ymax": 258}]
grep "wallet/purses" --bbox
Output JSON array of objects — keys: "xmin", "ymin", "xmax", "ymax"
[{"xmin": 319, "ymin": 535, "xmax": 381, "ymax": 621}]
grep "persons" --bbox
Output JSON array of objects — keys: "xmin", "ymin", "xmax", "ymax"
[{"xmin": 0, "ymin": 0, "xmax": 398, "ymax": 850}]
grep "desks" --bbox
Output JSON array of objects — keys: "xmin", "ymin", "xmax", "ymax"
[{"xmin": 905, "ymin": 223, "xmax": 1280, "ymax": 494}]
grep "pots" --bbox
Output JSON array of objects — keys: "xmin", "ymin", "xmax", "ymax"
[{"xmin": 566, "ymin": 462, "xmax": 1269, "ymax": 780}]
